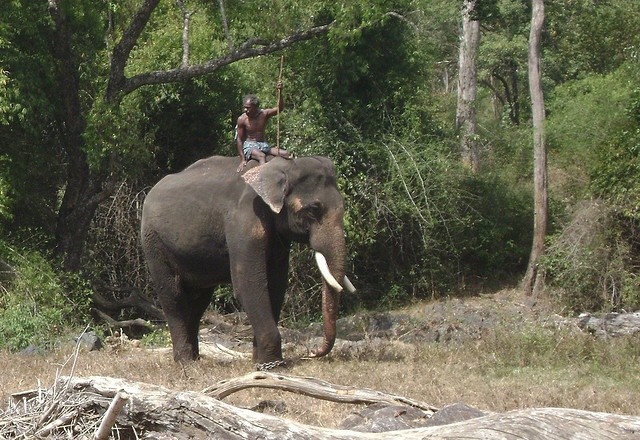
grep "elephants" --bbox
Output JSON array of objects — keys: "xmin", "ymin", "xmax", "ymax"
[{"xmin": 141, "ymin": 155, "xmax": 356, "ymax": 374}]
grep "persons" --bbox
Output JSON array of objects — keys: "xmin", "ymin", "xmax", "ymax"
[{"xmin": 236, "ymin": 80, "xmax": 294, "ymax": 174}]
